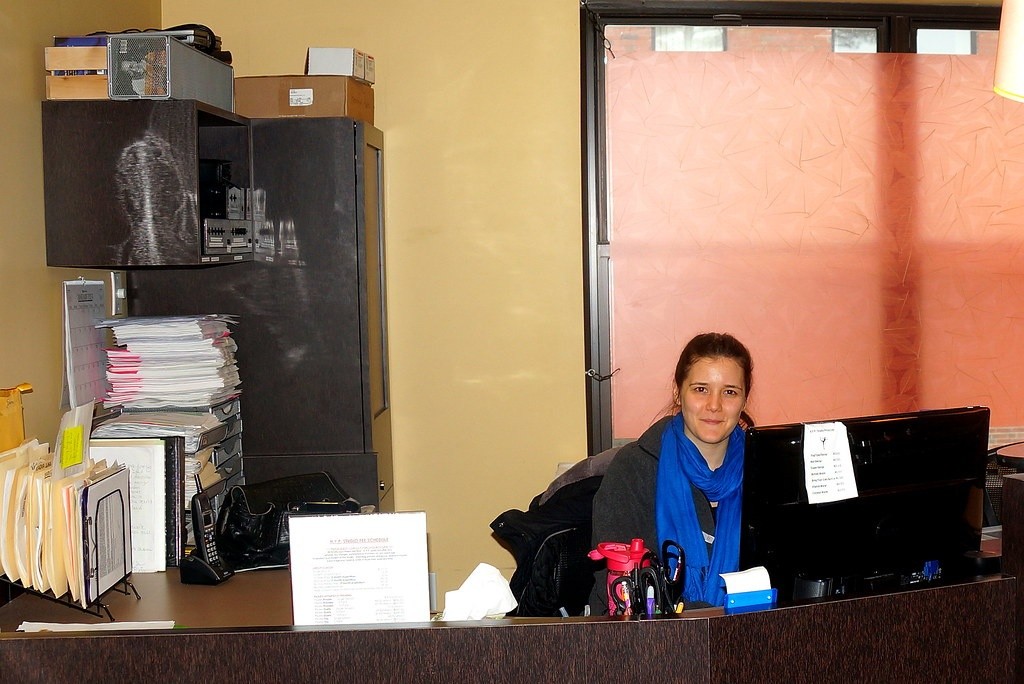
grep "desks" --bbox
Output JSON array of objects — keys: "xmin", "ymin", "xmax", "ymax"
[{"xmin": 0, "ymin": 554, "xmax": 293, "ymax": 636}]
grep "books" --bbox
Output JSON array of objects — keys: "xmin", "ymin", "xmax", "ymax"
[{"xmin": 0, "ymin": 312, "xmax": 244, "ymax": 609}]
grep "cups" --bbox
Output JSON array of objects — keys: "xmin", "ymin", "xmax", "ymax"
[{"xmin": 588, "ymin": 538, "xmax": 650, "ymax": 616}]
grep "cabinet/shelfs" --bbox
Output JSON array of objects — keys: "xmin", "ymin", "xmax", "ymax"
[
  {"xmin": 41, "ymin": 97, "xmax": 254, "ymax": 270},
  {"xmin": 126, "ymin": 120, "xmax": 395, "ymax": 514}
]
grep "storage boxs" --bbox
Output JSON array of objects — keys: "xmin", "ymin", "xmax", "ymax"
[
  {"xmin": 234, "ymin": 73, "xmax": 374, "ymax": 125},
  {"xmin": 304, "ymin": 45, "xmax": 376, "ymax": 86}
]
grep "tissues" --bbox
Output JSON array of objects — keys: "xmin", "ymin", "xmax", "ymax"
[
  {"xmin": 718, "ymin": 565, "xmax": 777, "ymax": 615},
  {"xmin": 430, "ymin": 562, "xmax": 518, "ymax": 621}
]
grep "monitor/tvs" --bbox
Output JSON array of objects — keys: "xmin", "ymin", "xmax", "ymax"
[{"xmin": 740, "ymin": 406, "xmax": 991, "ymax": 606}]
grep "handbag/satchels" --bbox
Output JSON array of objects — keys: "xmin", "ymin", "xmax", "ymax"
[{"xmin": 214, "ymin": 470, "xmax": 362, "ymax": 570}]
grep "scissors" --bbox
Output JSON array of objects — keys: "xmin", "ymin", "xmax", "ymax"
[{"xmin": 610, "ymin": 540, "xmax": 687, "ymax": 615}]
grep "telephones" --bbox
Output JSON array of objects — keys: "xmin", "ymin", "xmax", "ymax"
[{"xmin": 179, "ymin": 474, "xmax": 236, "ymax": 586}]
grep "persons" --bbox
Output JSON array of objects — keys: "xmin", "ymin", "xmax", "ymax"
[{"xmin": 578, "ymin": 330, "xmax": 755, "ymax": 617}]
